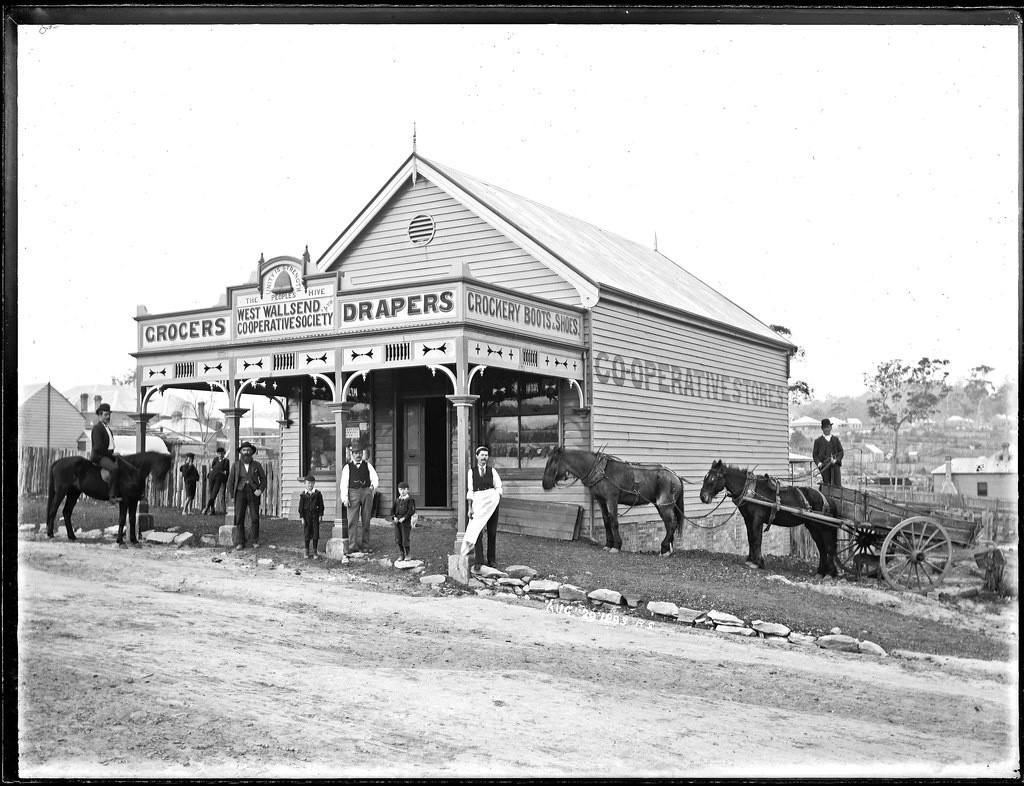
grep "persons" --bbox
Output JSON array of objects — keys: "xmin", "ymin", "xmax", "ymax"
[
  {"xmin": 340, "ymin": 448, "xmax": 379, "ymax": 553},
  {"xmin": 467, "ymin": 447, "xmax": 503, "ymax": 571},
  {"xmin": 180, "ymin": 453, "xmax": 199, "ymax": 515},
  {"xmin": 299, "ymin": 475, "xmax": 324, "ymax": 559},
  {"xmin": 813, "ymin": 419, "xmax": 844, "ymax": 485},
  {"xmin": 228, "ymin": 442, "xmax": 266, "ymax": 550},
  {"xmin": 91, "ymin": 403, "xmax": 120, "ymax": 505},
  {"xmin": 391, "ymin": 482, "xmax": 415, "ymax": 561},
  {"xmin": 202, "ymin": 448, "xmax": 229, "ymax": 515}
]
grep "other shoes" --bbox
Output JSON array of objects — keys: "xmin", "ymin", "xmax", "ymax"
[
  {"xmin": 252, "ymin": 541, "xmax": 260, "ymax": 548},
  {"xmin": 344, "ymin": 548, "xmax": 358, "ymax": 555},
  {"xmin": 474, "ymin": 563, "xmax": 482, "ymax": 570},
  {"xmin": 109, "ymin": 496, "xmax": 122, "ymax": 503},
  {"xmin": 398, "ymin": 554, "xmax": 405, "ymax": 560},
  {"xmin": 202, "ymin": 510, "xmax": 207, "ymax": 514},
  {"xmin": 236, "ymin": 543, "xmax": 244, "ymax": 549},
  {"xmin": 404, "ymin": 554, "xmax": 411, "ymax": 561},
  {"xmin": 208, "ymin": 510, "xmax": 215, "ymax": 514},
  {"xmin": 187, "ymin": 512, "xmax": 195, "ymax": 515},
  {"xmin": 488, "ymin": 560, "xmax": 496, "ymax": 567},
  {"xmin": 181, "ymin": 511, "xmax": 187, "ymax": 515},
  {"xmin": 313, "ymin": 553, "xmax": 318, "ymax": 559},
  {"xmin": 304, "ymin": 553, "xmax": 309, "ymax": 558}
]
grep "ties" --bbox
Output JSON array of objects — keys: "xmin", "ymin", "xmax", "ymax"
[{"xmin": 480, "ymin": 467, "xmax": 484, "ymax": 479}]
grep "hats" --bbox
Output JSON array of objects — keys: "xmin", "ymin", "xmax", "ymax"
[
  {"xmin": 821, "ymin": 419, "xmax": 833, "ymax": 427},
  {"xmin": 96, "ymin": 403, "xmax": 112, "ymax": 415},
  {"xmin": 350, "ymin": 444, "xmax": 365, "ymax": 452},
  {"xmin": 475, "ymin": 446, "xmax": 488, "ymax": 453},
  {"xmin": 237, "ymin": 442, "xmax": 256, "ymax": 455}
]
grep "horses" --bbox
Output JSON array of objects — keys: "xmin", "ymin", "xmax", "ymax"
[
  {"xmin": 44, "ymin": 451, "xmax": 173, "ymax": 549},
  {"xmin": 699, "ymin": 459, "xmax": 840, "ymax": 579},
  {"xmin": 542, "ymin": 446, "xmax": 684, "ymax": 557}
]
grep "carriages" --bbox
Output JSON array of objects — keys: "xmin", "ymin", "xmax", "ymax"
[{"xmin": 540, "ymin": 444, "xmax": 987, "ymax": 601}]
